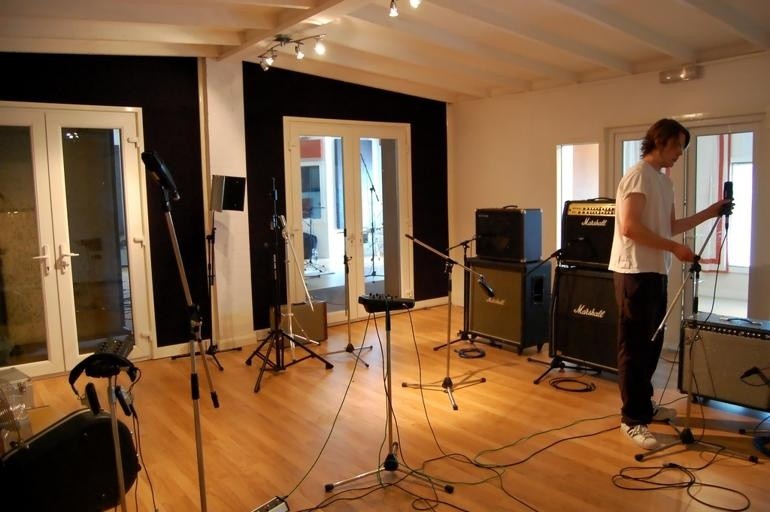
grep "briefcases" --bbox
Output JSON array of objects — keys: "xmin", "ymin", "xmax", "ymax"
[{"xmin": 271, "ymin": 301, "xmax": 327, "ymax": 348}]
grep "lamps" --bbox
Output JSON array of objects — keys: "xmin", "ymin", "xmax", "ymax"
[
  {"xmin": 256, "ymin": 32, "xmax": 328, "ymax": 72},
  {"xmin": 389, "ymin": 0, "xmax": 423, "ymax": 17},
  {"xmin": 659, "ymin": 66, "xmax": 704, "ymax": 84}
]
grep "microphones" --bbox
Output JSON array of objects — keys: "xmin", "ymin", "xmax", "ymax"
[
  {"xmin": 141, "ymin": 151, "xmax": 181, "ymax": 202},
  {"xmin": 724, "ymin": 182, "xmax": 733, "ymax": 233},
  {"xmin": 444, "ymin": 235, "xmax": 480, "ymax": 250}
]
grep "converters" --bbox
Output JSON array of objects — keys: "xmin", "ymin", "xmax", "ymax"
[{"xmin": 250, "ymin": 494, "xmax": 290, "ymax": 512}]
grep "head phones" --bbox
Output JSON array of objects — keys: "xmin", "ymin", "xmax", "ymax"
[{"xmin": 69, "ymin": 353, "xmax": 141, "ymax": 417}]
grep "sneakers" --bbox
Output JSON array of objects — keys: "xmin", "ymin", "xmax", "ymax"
[
  {"xmin": 650, "ymin": 401, "xmax": 677, "ymax": 420},
  {"xmin": 621, "ymin": 422, "xmax": 656, "ymax": 449}
]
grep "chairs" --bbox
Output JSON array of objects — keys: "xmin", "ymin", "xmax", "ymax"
[{"xmin": 0, "ymin": 407, "xmax": 141, "ymax": 512}]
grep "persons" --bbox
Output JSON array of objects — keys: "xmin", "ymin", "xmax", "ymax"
[{"xmin": 605, "ymin": 116, "xmax": 737, "ymax": 450}]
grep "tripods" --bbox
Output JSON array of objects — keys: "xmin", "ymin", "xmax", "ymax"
[
  {"xmin": 171, "ymin": 235, "xmax": 243, "ymax": 370},
  {"xmin": 402, "ymin": 263, "xmax": 486, "ymax": 410},
  {"xmin": 324, "ymin": 310, "xmax": 454, "ymax": 494},
  {"xmin": 245, "ymin": 223, "xmax": 333, "ymax": 392},
  {"xmin": 634, "ymin": 265, "xmax": 758, "ymax": 462},
  {"xmin": 433, "ymin": 246, "xmax": 503, "ymax": 352},
  {"xmin": 319, "ymin": 228, "xmax": 373, "ymax": 367},
  {"xmin": 527, "ymin": 357, "xmax": 600, "ymax": 384}
]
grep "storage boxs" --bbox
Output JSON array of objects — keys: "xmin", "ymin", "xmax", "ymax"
[{"xmin": 0, "ymin": 368, "xmax": 34, "ymax": 455}]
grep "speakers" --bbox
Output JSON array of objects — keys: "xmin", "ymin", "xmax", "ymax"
[
  {"xmin": 466, "ymin": 258, "xmax": 552, "ymax": 355},
  {"xmin": 219, "ymin": 176, "xmax": 246, "ymax": 213},
  {"xmin": 552, "ymin": 267, "xmax": 619, "ymax": 374},
  {"xmin": 475, "ymin": 207, "xmax": 542, "ymax": 264}
]
